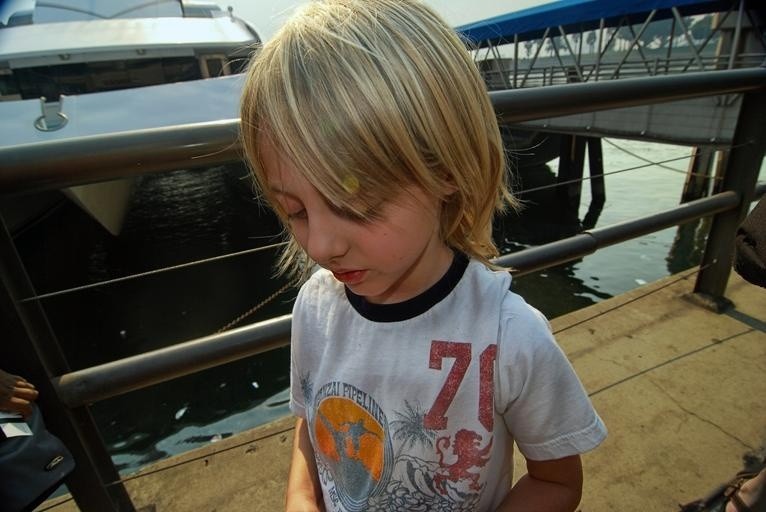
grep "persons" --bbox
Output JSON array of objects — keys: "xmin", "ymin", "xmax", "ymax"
[
  {"xmin": 239, "ymin": 1, "xmax": 608, "ymax": 512},
  {"xmin": 724, "ymin": 192, "xmax": 766, "ymax": 512},
  {"xmin": 0, "ymin": 369, "xmax": 40, "ymax": 421}
]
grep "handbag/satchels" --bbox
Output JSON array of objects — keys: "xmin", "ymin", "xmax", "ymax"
[{"xmin": 0, "ymin": 403, "xmax": 76, "ymax": 511}]
